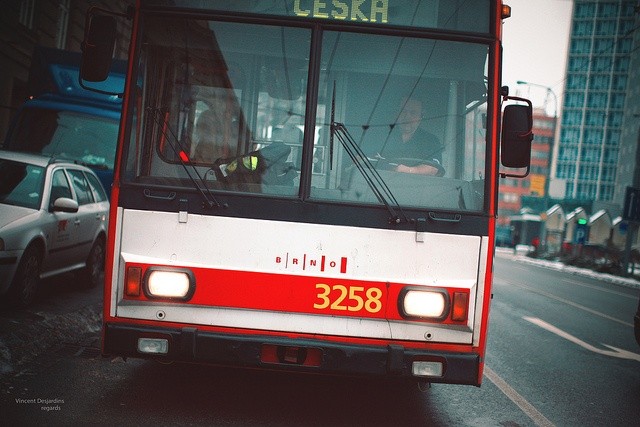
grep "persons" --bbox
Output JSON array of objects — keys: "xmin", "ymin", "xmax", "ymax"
[
  {"xmin": 191, "ymin": 110, "xmax": 230, "ymax": 163},
  {"xmin": 353, "ymin": 89, "xmax": 445, "ymax": 177}
]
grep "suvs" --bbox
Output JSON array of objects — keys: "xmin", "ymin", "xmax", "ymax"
[{"xmin": 0, "ymin": 150, "xmax": 110, "ymax": 310}]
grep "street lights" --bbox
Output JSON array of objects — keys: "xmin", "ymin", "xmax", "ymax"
[{"xmin": 517, "ymin": 80, "xmax": 556, "ymax": 258}]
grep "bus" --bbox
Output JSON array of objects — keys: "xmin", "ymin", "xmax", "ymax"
[{"xmin": 79, "ymin": 0, "xmax": 533, "ymax": 387}]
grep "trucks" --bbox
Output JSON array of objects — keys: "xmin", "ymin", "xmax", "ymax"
[{"xmin": 7, "ymin": 67, "xmax": 125, "ymax": 199}]
